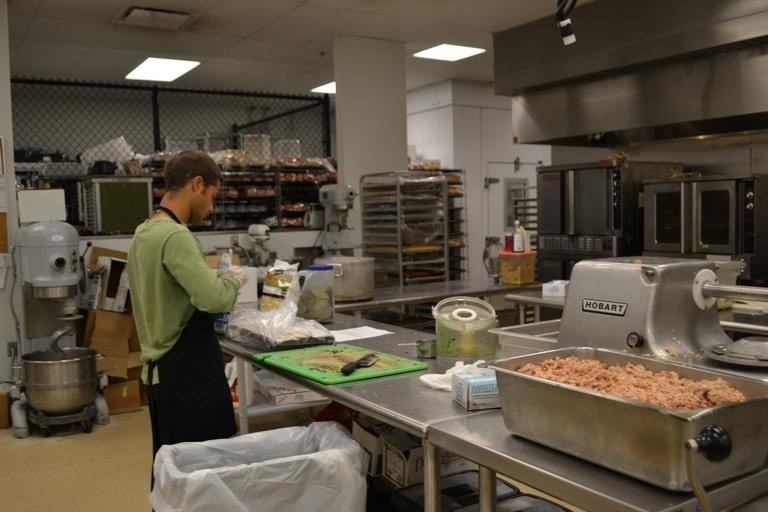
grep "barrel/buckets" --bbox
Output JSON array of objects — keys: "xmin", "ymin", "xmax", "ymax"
[{"xmin": 295, "ymin": 265, "xmax": 335, "ymax": 324}]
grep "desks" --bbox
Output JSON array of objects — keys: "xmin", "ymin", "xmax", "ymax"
[
  {"xmin": 428, "ymin": 411, "xmax": 767, "ymax": 512},
  {"xmin": 217, "ymin": 313, "xmax": 500, "ymax": 512},
  {"xmin": 504, "ymin": 288, "xmax": 565, "ymax": 324},
  {"xmin": 336, "ymin": 279, "xmax": 567, "ymax": 333}
]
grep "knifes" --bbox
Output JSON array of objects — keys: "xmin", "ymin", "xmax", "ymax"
[{"xmin": 341, "ymin": 353, "xmax": 377, "ymax": 374}]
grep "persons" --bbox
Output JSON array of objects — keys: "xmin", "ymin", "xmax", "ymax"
[{"xmin": 126, "ymin": 149, "xmax": 250, "ymax": 512}]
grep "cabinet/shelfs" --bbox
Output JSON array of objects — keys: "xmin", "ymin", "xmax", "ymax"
[
  {"xmin": 511, "ymin": 184, "xmax": 538, "ymax": 322},
  {"xmin": 361, "ymin": 167, "xmax": 450, "ymax": 284},
  {"xmin": 143, "ymin": 152, "xmax": 339, "ymax": 231},
  {"xmin": 442, "ymin": 168, "xmax": 469, "ymax": 281}
]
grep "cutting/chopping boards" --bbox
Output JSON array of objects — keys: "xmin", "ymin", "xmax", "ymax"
[{"xmin": 254, "ymin": 342, "xmax": 428, "ymax": 385}]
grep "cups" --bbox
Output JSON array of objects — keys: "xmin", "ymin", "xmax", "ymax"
[
  {"xmin": 303, "ymin": 202, "xmax": 323, "ymax": 230},
  {"xmin": 417, "ymin": 339, "xmax": 436, "ymax": 358}
]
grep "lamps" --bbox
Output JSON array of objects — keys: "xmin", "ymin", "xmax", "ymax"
[{"xmin": 554, "ymin": 0, "xmax": 578, "ymax": 46}]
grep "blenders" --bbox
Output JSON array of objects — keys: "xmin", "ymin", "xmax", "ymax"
[
  {"xmin": 248, "ymin": 223, "xmax": 270, "ymax": 265},
  {"xmin": 318, "ymin": 183, "xmax": 358, "ymax": 235},
  {"xmin": 14, "ymin": 221, "xmax": 98, "ymax": 436}
]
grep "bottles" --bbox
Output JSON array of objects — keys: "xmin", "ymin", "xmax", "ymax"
[
  {"xmin": 39, "ymin": 156, "xmax": 54, "ymax": 189},
  {"xmin": 511, "ymin": 220, "xmax": 525, "ymax": 252},
  {"xmin": 504, "ymin": 235, "xmax": 512, "ymax": 253}
]
